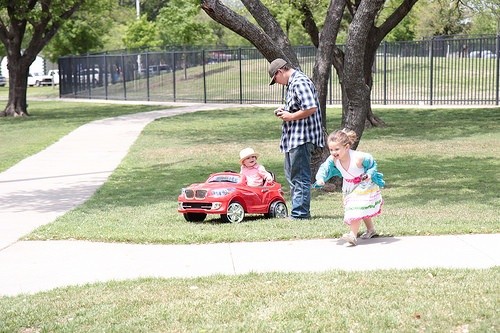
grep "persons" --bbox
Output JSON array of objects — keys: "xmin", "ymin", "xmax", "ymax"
[
  {"xmin": 238, "ymin": 148, "xmax": 273, "ymax": 187},
  {"xmin": 314, "ymin": 129, "xmax": 385, "ymax": 245},
  {"xmin": 268, "ymin": 58, "xmax": 322, "ymax": 220}
]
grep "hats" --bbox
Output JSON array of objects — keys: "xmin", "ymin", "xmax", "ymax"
[
  {"xmin": 238, "ymin": 148, "xmax": 259, "ymax": 163},
  {"xmin": 268, "ymin": 58, "xmax": 287, "ymax": 86}
]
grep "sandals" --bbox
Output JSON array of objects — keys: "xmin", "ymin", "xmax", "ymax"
[
  {"xmin": 344, "ymin": 234, "xmax": 358, "ymax": 246},
  {"xmin": 361, "ymin": 229, "xmax": 377, "ymax": 239}
]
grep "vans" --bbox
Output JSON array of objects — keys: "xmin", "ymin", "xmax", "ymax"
[{"xmin": 148, "ymin": 65, "xmax": 169, "ymax": 74}]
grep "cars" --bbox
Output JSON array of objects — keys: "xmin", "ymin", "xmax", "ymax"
[
  {"xmin": 139, "ymin": 69, "xmax": 152, "ymax": 78},
  {"xmin": 177, "ymin": 170, "xmax": 288, "ymax": 224},
  {"xmin": 110, "ymin": 68, "xmax": 134, "ymax": 83},
  {"xmin": 482, "ymin": 49, "xmax": 495, "ymax": 59},
  {"xmin": 70, "ymin": 68, "xmax": 112, "ymax": 85},
  {"xmin": 0, "ymin": 74, "xmax": 6, "ymax": 88},
  {"xmin": 48, "ymin": 69, "xmax": 78, "ymax": 86},
  {"xmin": 470, "ymin": 51, "xmax": 482, "ymax": 58},
  {"xmin": 27, "ymin": 71, "xmax": 52, "ymax": 88}
]
